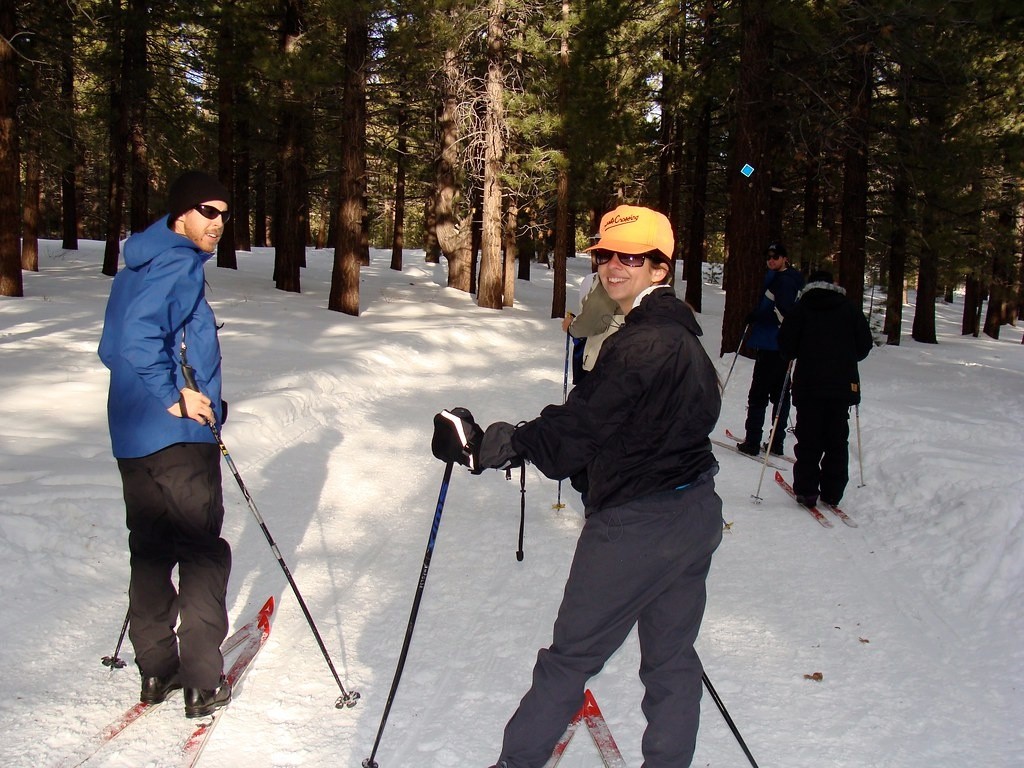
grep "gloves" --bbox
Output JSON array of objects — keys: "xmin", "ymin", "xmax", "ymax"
[{"xmin": 432, "ymin": 409, "xmax": 485, "ymax": 474}]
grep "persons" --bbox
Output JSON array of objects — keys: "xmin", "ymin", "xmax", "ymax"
[
  {"xmin": 737, "ymin": 242, "xmax": 807, "ymax": 455},
  {"xmin": 98, "ymin": 170, "xmax": 233, "ymax": 720},
  {"xmin": 431, "ymin": 202, "xmax": 722, "ymax": 768},
  {"xmin": 776, "ymin": 269, "xmax": 874, "ymax": 509}
]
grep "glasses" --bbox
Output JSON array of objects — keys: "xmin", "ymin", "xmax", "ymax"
[
  {"xmin": 594, "ymin": 248, "xmax": 661, "ymax": 268},
  {"xmin": 192, "ymin": 204, "xmax": 230, "ymax": 225},
  {"xmin": 765, "ymin": 253, "xmax": 785, "ymax": 263}
]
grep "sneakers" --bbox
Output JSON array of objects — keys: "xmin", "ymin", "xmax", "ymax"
[
  {"xmin": 736, "ymin": 440, "xmax": 760, "ymax": 456},
  {"xmin": 796, "ymin": 495, "xmax": 818, "ymax": 509},
  {"xmin": 764, "ymin": 443, "xmax": 783, "ymax": 456},
  {"xmin": 820, "ymin": 494, "xmax": 838, "ymax": 505}
]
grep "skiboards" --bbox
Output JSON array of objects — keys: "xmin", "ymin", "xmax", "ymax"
[
  {"xmin": 773, "ymin": 469, "xmax": 859, "ymax": 529},
  {"xmin": 540, "ymin": 686, "xmax": 629, "ymax": 768},
  {"xmin": 55, "ymin": 593, "xmax": 276, "ymax": 768},
  {"xmin": 709, "ymin": 427, "xmax": 797, "ymax": 471}
]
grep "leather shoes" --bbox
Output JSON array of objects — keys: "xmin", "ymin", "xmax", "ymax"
[
  {"xmin": 140, "ymin": 673, "xmax": 185, "ymax": 705},
  {"xmin": 184, "ymin": 680, "xmax": 232, "ymax": 718}
]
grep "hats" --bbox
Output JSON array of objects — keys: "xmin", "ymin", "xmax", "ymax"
[
  {"xmin": 765, "ymin": 245, "xmax": 789, "ymax": 260},
  {"xmin": 167, "ymin": 172, "xmax": 230, "ymax": 230},
  {"xmin": 808, "ymin": 271, "xmax": 834, "ymax": 284},
  {"xmin": 580, "ymin": 204, "xmax": 675, "ymax": 260}
]
